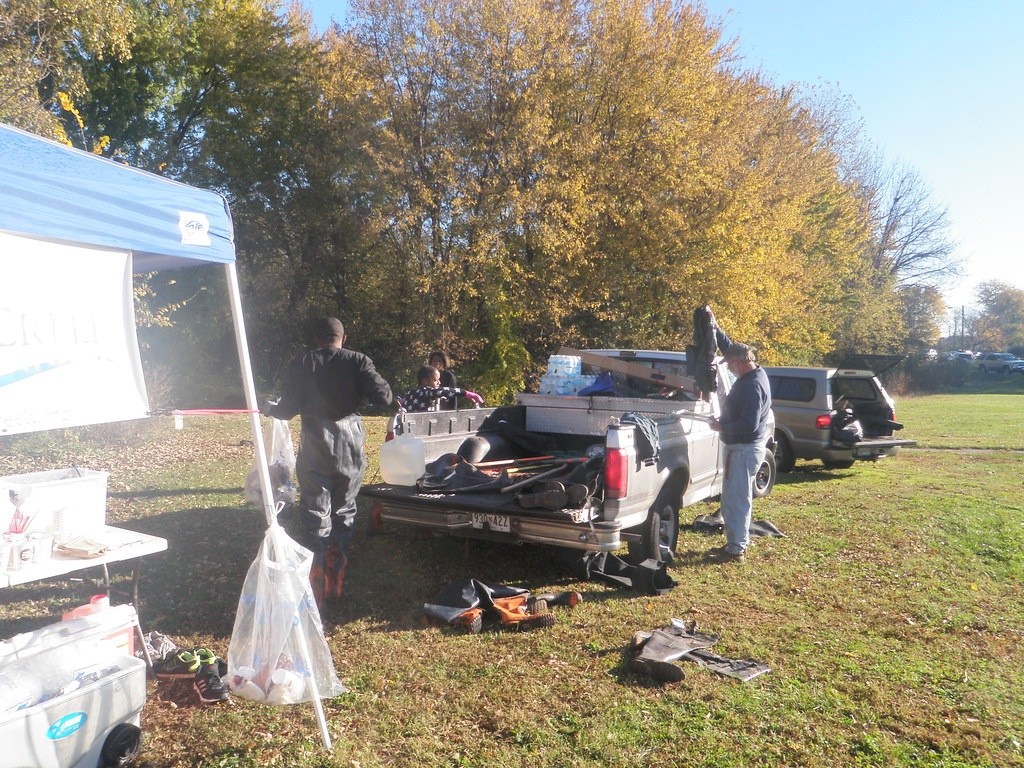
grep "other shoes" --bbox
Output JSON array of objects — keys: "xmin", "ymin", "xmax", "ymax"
[{"xmin": 711, "ymin": 546, "xmax": 747, "ymax": 562}]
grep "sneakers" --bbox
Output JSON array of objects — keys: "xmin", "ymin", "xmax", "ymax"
[
  {"xmin": 305, "ymin": 543, "xmax": 348, "ymax": 617},
  {"xmin": 152, "ymin": 646, "xmax": 215, "ymax": 679},
  {"xmin": 194, "ymin": 658, "xmax": 230, "ymax": 702}
]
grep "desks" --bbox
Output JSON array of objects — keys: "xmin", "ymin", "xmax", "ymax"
[{"xmin": 0, "ymin": 526, "xmax": 168, "ymax": 679}]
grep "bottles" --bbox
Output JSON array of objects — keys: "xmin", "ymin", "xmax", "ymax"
[{"xmin": 539, "ymin": 355, "xmax": 598, "ymax": 397}]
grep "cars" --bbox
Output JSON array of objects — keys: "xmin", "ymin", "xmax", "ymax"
[
  {"xmin": 924, "ymin": 349, "xmax": 972, "ymax": 364},
  {"xmin": 760, "ymin": 355, "xmax": 918, "ymax": 475},
  {"xmin": 975, "ymin": 353, "xmax": 1024, "ymax": 375}
]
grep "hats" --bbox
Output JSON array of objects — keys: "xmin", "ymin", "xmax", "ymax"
[{"xmin": 718, "ymin": 343, "xmax": 755, "ymax": 364}]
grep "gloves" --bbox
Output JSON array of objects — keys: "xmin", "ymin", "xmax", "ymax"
[{"xmin": 466, "ymin": 390, "xmax": 484, "ymax": 404}]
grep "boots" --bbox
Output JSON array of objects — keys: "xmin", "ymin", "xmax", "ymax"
[
  {"xmin": 517, "ymin": 452, "xmax": 604, "ymax": 511},
  {"xmin": 424, "ymin": 579, "xmax": 583, "ymax": 634}
]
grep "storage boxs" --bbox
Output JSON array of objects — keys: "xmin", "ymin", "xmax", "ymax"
[{"xmin": 0, "ymin": 468, "xmax": 111, "ymax": 547}]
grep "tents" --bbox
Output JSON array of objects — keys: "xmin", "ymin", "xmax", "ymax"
[{"xmin": 0, "ymin": 122, "xmax": 333, "ymax": 753}]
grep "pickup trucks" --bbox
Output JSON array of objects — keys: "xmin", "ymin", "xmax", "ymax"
[{"xmin": 356, "ymin": 346, "xmax": 776, "ymax": 567}]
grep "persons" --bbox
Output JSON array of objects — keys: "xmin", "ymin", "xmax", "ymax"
[
  {"xmin": 261, "ymin": 317, "xmax": 393, "ymax": 609},
  {"xmin": 401, "ymin": 350, "xmax": 484, "ymax": 413},
  {"xmin": 700, "ymin": 305, "xmax": 771, "ymax": 562}
]
